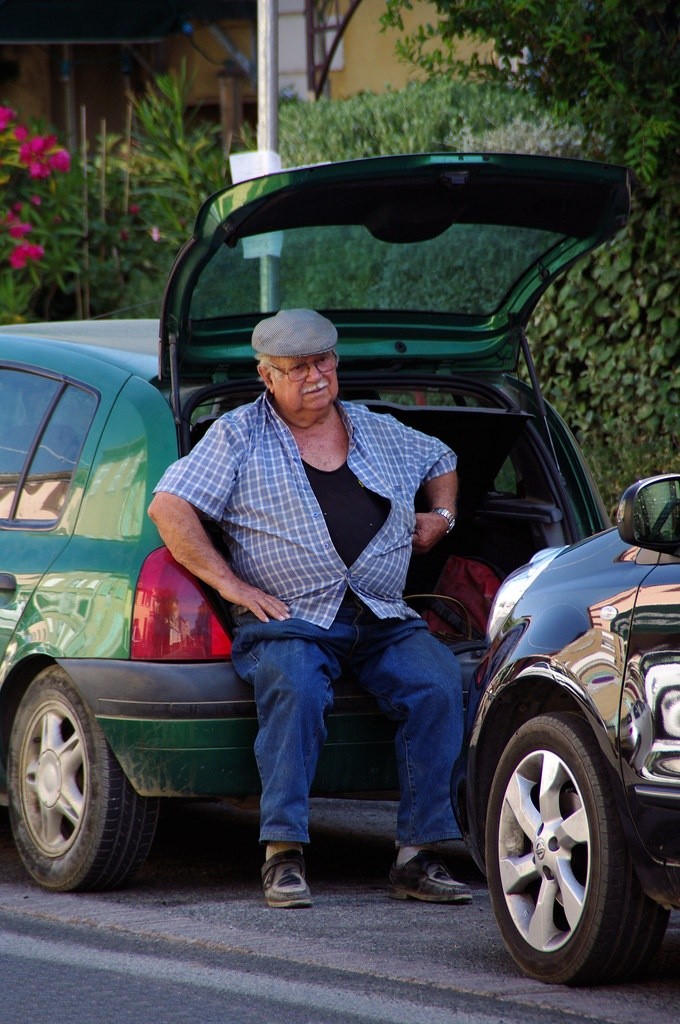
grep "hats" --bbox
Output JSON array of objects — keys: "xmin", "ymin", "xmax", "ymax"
[{"xmin": 252, "ymin": 309, "xmax": 338, "ymax": 357}]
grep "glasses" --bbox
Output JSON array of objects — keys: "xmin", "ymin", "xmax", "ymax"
[{"xmin": 271, "ymin": 351, "xmax": 338, "ymax": 382}]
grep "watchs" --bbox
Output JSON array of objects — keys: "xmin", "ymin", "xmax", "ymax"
[{"xmin": 431, "ymin": 508, "xmax": 455, "ymax": 534}]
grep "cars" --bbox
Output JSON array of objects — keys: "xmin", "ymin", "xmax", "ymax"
[
  {"xmin": 449, "ymin": 475, "xmax": 680, "ymax": 987},
  {"xmin": 0, "ymin": 154, "xmax": 632, "ymax": 892}
]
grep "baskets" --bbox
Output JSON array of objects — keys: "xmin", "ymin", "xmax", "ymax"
[{"xmin": 402, "ymin": 594, "xmax": 471, "ymax": 647}]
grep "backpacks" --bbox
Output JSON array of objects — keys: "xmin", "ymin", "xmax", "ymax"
[{"xmin": 420, "ymin": 554, "xmax": 504, "ymax": 644}]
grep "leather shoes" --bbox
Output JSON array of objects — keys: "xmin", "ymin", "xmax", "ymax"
[
  {"xmin": 387, "ymin": 849, "xmax": 473, "ymax": 902},
  {"xmin": 261, "ymin": 849, "xmax": 314, "ymax": 908}
]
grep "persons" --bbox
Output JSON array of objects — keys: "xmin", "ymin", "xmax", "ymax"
[{"xmin": 146, "ymin": 307, "xmax": 473, "ymax": 909}]
